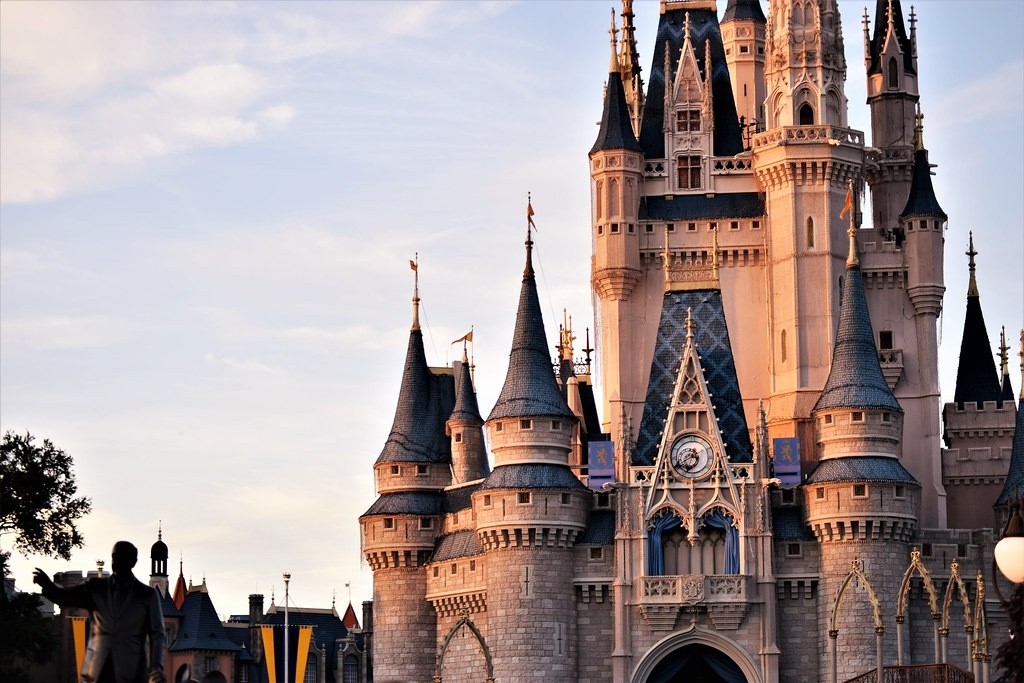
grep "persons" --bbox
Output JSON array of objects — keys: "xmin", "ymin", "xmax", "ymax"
[{"xmin": 32, "ymin": 541, "xmax": 167, "ymax": 683}]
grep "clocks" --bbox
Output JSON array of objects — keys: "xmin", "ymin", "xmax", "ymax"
[{"xmin": 671, "ymin": 435, "xmax": 714, "ymax": 478}]
